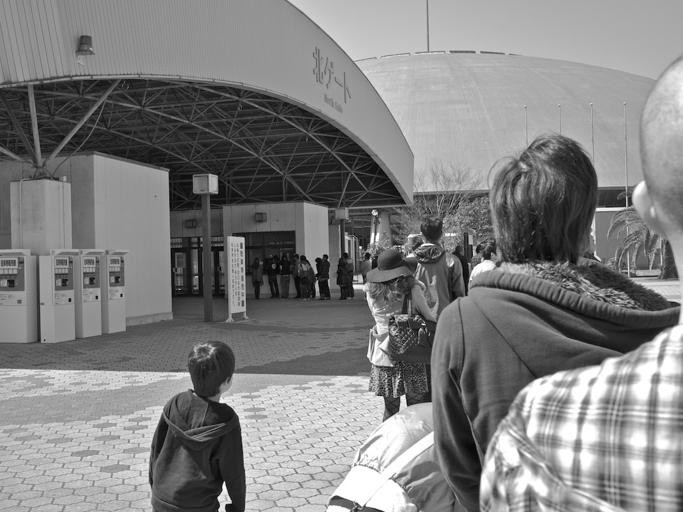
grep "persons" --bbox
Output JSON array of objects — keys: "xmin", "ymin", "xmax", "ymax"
[
  {"xmin": 477, "ymin": 53, "xmax": 683, "ymax": 512},
  {"xmin": 248, "ymin": 251, "xmax": 356, "ymax": 303},
  {"xmin": 429, "ymin": 129, "xmax": 682, "ymax": 511},
  {"xmin": 360, "ymin": 219, "xmax": 503, "ymax": 422},
  {"xmin": 584, "ymin": 233, "xmax": 598, "ymax": 258},
  {"xmin": 149, "ymin": 339, "xmax": 246, "ymax": 511},
  {"xmin": 323, "ymin": 401, "xmax": 472, "ymax": 512}
]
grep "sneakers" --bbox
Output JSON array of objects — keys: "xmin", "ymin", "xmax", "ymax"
[{"xmin": 254, "ymin": 294, "xmax": 358, "ymax": 301}]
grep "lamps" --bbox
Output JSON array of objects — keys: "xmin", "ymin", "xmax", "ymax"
[{"xmin": 74, "ymin": 32, "xmax": 95, "ymax": 58}]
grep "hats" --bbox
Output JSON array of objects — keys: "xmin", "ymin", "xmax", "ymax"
[{"xmin": 364, "ymin": 248, "xmax": 412, "ymax": 284}]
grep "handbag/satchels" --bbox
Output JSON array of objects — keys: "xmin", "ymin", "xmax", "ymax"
[{"xmin": 386, "ymin": 284, "xmax": 438, "ymax": 363}]
